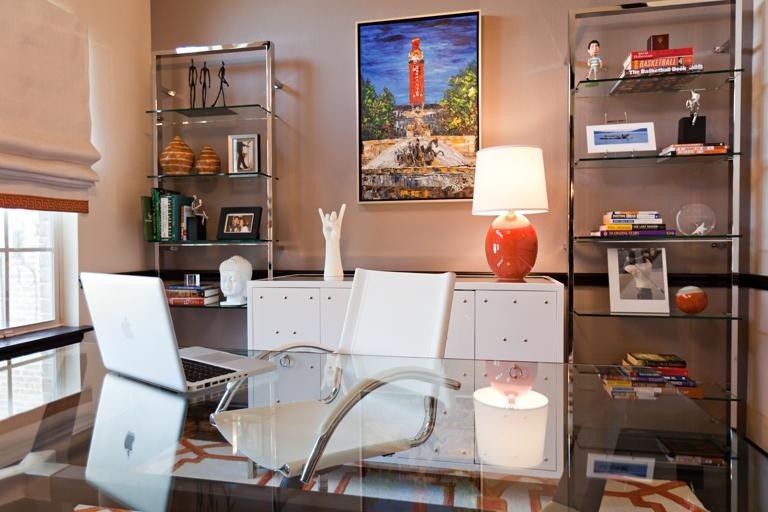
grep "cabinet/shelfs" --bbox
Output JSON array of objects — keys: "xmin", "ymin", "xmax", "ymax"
[
  {"xmin": 326, "ymin": 288, "xmax": 472, "ymax": 464},
  {"xmin": 565, "ymin": 0, "xmax": 754, "ymax": 461},
  {"xmin": 142, "ymin": 41, "xmax": 285, "ymax": 312},
  {"xmin": 249, "ymin": 284, "xmax": 321, "ymax": 410},
  {"xmin": 474, "ymin": 291, "xmax": 560, "ymax": 475}
]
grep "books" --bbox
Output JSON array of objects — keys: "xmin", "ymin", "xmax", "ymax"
[
  {"xmin": 591, "ymin": 210, "xmax": 675, "ymax": 237},
  {"xmin": 609, "ymin": 47, "xmax": 704, "ymax": 97},
  {"xmin": 656, "ymin": 142, "xmax": 730, "ymax": 164},
  {"xmin": 596, "ymin": 353, "xmax": 704, "ymax": 400},
  {"xmin": 656, "ymin": 438, "xmax": 726, "ymax": 467},
  {"xmin": 150, "ymin": 188, "xmax": 207, "ymax": 241},
  {"xmin": 163, "ymin": 282, "xmax": 219, "ymax": 305}
]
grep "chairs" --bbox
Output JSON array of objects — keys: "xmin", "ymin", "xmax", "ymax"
[{"xmin": 208, "ymin": 266, "xmax": 462, "ymax": 499}]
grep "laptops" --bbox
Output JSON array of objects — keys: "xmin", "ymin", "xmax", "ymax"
[
  {"xmin": 86, "ymin": 373, "xmax": 255, "ymax": 512},
  {"xmin": 79, "ymin": 269, "xmax": 277, "ymax": 393}
]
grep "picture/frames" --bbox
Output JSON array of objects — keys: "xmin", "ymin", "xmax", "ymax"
[
  {"xmin": 218, "ymin": 203, "xmax": 261, "ymax": 240},
  {"xmin": 352, "ymin": 7, "xmax": 484, "ymax": 206},
  {"xmin": 586, "ymin": 451, "xmax": 658, "ymax": 485},
  {"xmin": 604, "ymin": 242, "xmax": 671, "ymax": 313},
  {"xmin": 227, "ymin": 132, "xmax": 259, "ymax": 179},
  {"xmin": 585, "ymin": 122, "xmax": 660, "ymax": 155}
]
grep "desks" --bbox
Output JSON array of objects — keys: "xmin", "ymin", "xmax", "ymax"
[{"xmin": 0, "ymin": 337, "xmax": 768, "ymax": 510}]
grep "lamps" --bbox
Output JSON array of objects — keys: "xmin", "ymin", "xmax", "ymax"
[
  {"xmin": 467, "ymin": 145, "xmax": 554, "ymax": 280},
  {"xmin": 465, "ymin": 342, "xmax": 551, "ymax": 471}
]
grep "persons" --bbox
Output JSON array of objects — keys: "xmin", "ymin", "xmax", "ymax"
[
  {"xmin": 316, "ymin": 203, "xmax": 352, "ymax": 283},
  {"xmin": 236, "ymin": 142, "xmax": 249, "ymax": 169},
  {"xmin": 586, "ymin": 40, "xmax": 603, "ymax": 81},
  {"xmin": 231, "ymin": 216, "xmax": 249, "ymax": 232},
  {"xmin": 220, "ymin": 253, "xmax": 252, "ymax": 308},
  {"xmin": 624, "ymin": 252, "xmax": 653, "ymax": 299}
]
